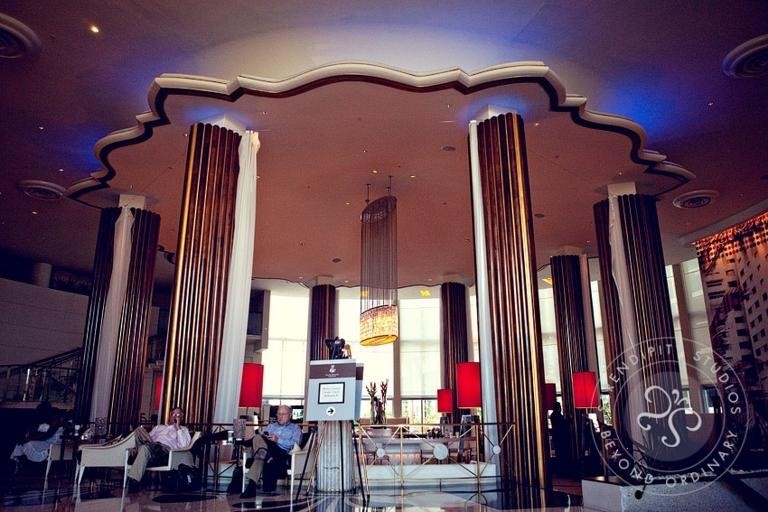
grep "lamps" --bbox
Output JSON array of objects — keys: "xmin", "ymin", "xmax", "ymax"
[
  {"xmin": 571, "ymin": 371, "xmax": 601, "ymax": 456},
  {"xmin": 436, "ymin": 361, "xmax": 486, "ymax": 463},
  {"xmin": 359, "ymin": 175, "xmax": 401, "ymax": 345},
  {"xmin": 545, "ymin": 384, "xmax": 557, "ymax": 408},
  {"xmin": 234, "ymin": 363, "xmax": 263, "ymax": 420}
]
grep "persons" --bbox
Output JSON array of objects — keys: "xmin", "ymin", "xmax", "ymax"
[
  {"xmin": 550, "ymin": 398, "xmax": 601, "ymax": 466},
  {"xmin": 123, "ymin": 407, "xmax": 193, "ymax": 498},
  {"xmin": 238, "ymin": 404, "xmax": 304, "ymax": 500}
]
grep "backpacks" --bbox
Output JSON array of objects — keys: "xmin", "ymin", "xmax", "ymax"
[
  {"xmin": 172, "ymin": 462, "xmax": 200, "ymax": 491},
  {"xmin": 227, "ymin": 466, "xmax": 244, "ymax": 493}
]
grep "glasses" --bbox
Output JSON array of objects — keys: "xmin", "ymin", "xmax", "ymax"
[{"xmin": 170, "ymin": 412, "xmax": 183, "ymax": 417}]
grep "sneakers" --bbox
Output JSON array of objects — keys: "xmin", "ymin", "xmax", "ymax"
[
  {"xmin": 128, "ymin": 484, "xmax": 139, "ymax": 493},
  {"xmin": 257, "ymin": 446, "xmax": 275, "ymax": 465},
  {"xmin": 239, "ymin": 489, "xmax": 257, "ymax": 499}
]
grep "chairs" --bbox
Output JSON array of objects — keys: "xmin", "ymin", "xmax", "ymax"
[
  {"xmin": 241, "ymin": 432, "xmax": 317, "ymax": 507},
  {"xmin": 12, "ymin": 424, "xmax": 205, "ymax": 504}
]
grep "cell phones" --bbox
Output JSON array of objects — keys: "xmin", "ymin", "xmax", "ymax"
[
  {"xmin": 264, "ymin": 431, "xmax": 269, "ymax": 436},
  {"xmin": 174, "ymin": 418, "xmax": 177, "ymax": 423}
]
